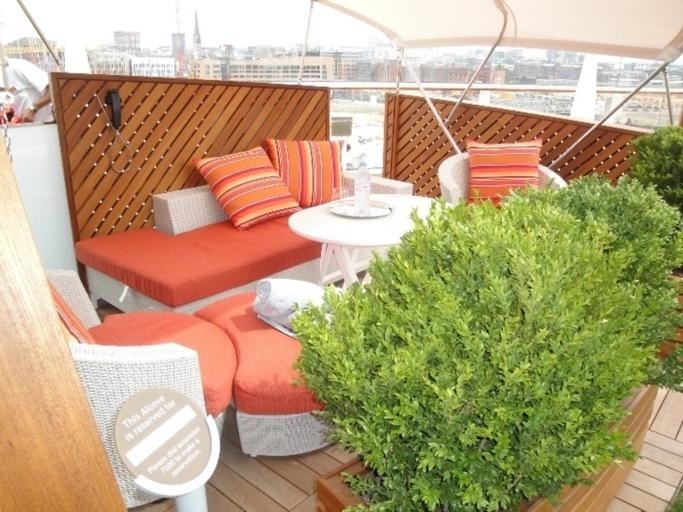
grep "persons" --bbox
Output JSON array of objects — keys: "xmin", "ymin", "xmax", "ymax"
[{"xmin": 0, "ymin": 55, "xmax": 55, "ymax": 123}]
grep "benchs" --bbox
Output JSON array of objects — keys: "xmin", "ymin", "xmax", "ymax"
[{"xmin": 72, "ymin": 171, "xmax": 413, "ymax": 314}]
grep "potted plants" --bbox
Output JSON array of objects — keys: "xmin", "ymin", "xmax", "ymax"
[{"xmin": 295, "ymin": 176, "xmax": 680, "ymax": 512}]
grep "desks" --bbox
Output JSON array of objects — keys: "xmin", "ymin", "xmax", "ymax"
[{"xmin": 290, "ymin": 193, "xmax": 444, "ymax": 292}]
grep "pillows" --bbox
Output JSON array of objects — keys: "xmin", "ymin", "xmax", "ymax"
[
  {"xmin": 468, "ymin": 140, "xmax": 544, "ymax": 207},
  {"xmin": 154, "ymin": 138, "xmax": 345, "ymax": 237}
]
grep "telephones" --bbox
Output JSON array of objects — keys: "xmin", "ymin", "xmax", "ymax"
[{"xmin": 105, "ymin": 91, "xmax": 122, "ymax": 129}]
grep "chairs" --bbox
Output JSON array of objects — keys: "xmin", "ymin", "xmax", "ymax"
[
  {"xmin": 438, "ymin": 152, "xmax": 568, "ymax": 209},
  {"xmin": 42, "ymin": 264, "xmax": 236, "ymax": 512}
]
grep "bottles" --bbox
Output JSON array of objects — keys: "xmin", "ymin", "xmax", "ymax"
[{"xmin": 353, "ymin": 161, "xmax": 371, "ymax": 214}]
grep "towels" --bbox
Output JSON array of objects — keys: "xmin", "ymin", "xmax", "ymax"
[{"xmin": 252, "ymin": 279, "xmax": 335, "ymax": 341}]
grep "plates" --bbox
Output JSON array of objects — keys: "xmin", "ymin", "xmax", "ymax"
[{"xmin": 330, "ymin": 200, "xmax": 393, "ymax": 219}]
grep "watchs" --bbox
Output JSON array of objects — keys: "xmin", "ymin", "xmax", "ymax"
[{"xmin": 27, "ymin": 103, "xmax": 37, "ymax": 114}]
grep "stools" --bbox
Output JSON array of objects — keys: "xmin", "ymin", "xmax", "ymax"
[{"xmin": 195, "ymin": 289, "xmax": 340, "ymax": 460}]
